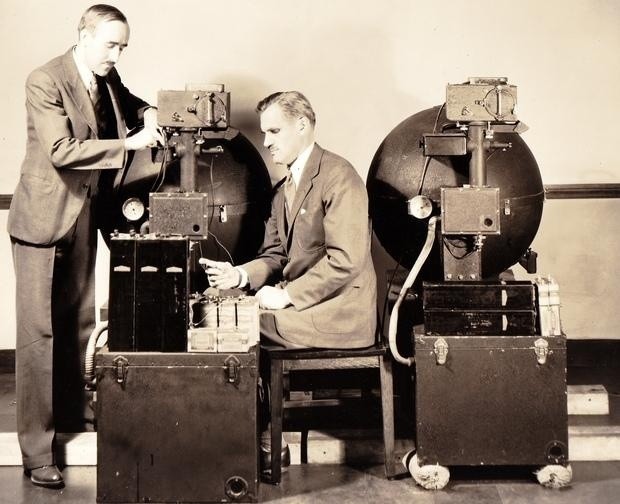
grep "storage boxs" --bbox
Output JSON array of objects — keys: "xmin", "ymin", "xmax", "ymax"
[
  {"xmin": 415, "ymin": 323, "xmax": 570, "ymax": 468},
  {"xmin": 95, "ymin": 345, "xmax": 258, "ymax": 504}
]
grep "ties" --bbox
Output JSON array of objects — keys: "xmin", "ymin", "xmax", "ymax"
[
  {"xmin": 284, "ymin": 169, "xmax": 297, "ymax": 229},
  {"xmin": 90, "ymin": 75, "xmax": 100, "ymax": 197}
]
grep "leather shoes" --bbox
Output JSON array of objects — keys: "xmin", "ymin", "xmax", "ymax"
[
  {"xmin": 260, "ymin": 446, "xmax": 290, "ymax": 474},
  {"xmin": 24, "ymin": 464, "xmax": 64, "ymax": 487}
]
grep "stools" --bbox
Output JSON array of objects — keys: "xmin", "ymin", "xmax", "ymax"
[{"xmin": 259, "ymin": 340, "xmax": 398, "ymax": 485}]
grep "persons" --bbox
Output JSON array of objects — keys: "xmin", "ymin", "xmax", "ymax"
[
  {"xmin": 5, "ymin": 3, "xmax": 165, "ymax": 486},
  {"xmin": 199, "ymin": 89, "xmax": 378, "ymax": 473}
]
